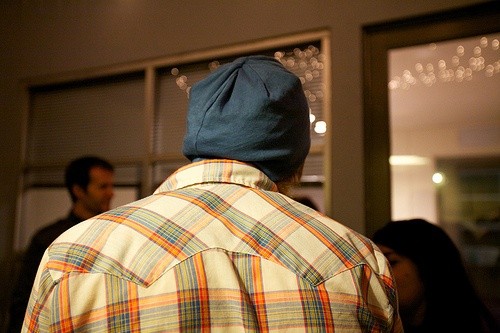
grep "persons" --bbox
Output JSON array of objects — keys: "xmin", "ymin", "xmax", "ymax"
[
  {"xmin": 17, "ymin": 54, "xmax": 405, "ymax": 333},
  {"xmin": 450, "ymin": 209, "xmax": 499, "ymax": 249},
  {"xmin": 26, "ymin": 155, "xmax": 117, "ymax": 298},
  {"xmin": 368, "ymin": 216, "xmax": 479, "ymax": 332}
]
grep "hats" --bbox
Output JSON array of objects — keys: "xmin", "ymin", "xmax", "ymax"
[{"xmin": 183, "ymin": 56, "xmax": 310, "ymax": 181}]
